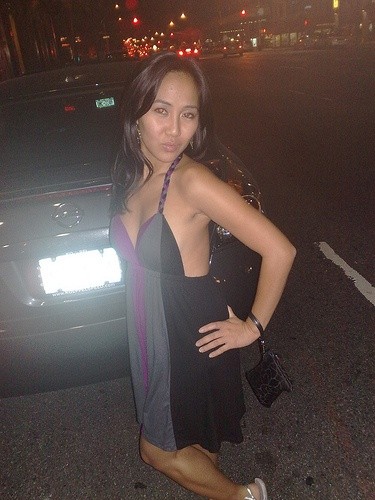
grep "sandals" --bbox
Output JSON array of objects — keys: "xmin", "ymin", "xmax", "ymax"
[{"xmin": 244, "ymin": 477, "xmax": 268, "ymax": 500}]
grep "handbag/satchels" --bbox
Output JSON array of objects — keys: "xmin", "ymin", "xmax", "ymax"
[{"xmin": 246, "ymin": 312, "xmax": 293, "ymax": 408}]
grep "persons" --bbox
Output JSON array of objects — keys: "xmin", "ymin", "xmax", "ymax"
[{"xmin": 107, "ymin": 51, "xmax": 299, "ymax": 500}]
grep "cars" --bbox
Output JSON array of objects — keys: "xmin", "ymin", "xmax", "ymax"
[
  {"xmin": 178, "ymin": 46, "xmax": 199, "ymax": 60},
  {"xmin": 0, "ymin": 60, "xmax": 266, "ymax": 400},
  {"xmin": 223, "ymin": 39, "xmax": 243, "ymax": 58},
  {"xmin": 127, "ymin": 45, "xmax": 148, "ymax": 58}
]
